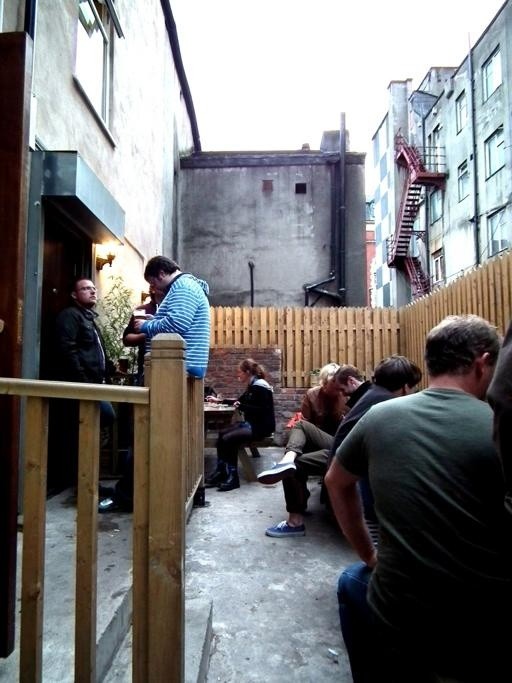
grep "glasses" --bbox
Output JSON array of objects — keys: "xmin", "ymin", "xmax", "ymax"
[{"xmin": 76, "ymin": 286, "xmax": 97, "ymax": 290}]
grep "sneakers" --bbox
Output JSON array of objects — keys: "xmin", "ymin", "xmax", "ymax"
[
  {"xmin": 99, "ymin": 497, "xmax": 133, "ymax": 513},
  {"xmin": 265, "ymin": 520, "xmax": 305, "ymax": 536},
  {"xmin": 257, "ymin": 462, "xmax": 296, "ymax": 484}
]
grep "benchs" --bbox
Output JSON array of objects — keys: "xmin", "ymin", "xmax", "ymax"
[{"xmin": 201, "ymin": 433, "xmax": 276, "ymax": 484}]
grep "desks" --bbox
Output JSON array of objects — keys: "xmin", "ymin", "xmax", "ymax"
[{"xmin": 205, "ymin": 394, "xmax": 237, "ymax": 432}]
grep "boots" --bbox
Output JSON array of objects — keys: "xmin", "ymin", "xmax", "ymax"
[
  {"xmin": 205, "ymin": 463, "xmax": 227, "ymax": 485},
  {"xmin": 216, "ymin": 466, "xmax": 240, "ymax": 489}
]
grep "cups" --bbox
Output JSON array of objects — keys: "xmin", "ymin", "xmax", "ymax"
[
  {"xmin": 133, "ymin": 309, "xmax": 146, "ymax": 320},
  {"xmin": 118, "ymin": 356, "xmax": 129, "ymax": 373}
]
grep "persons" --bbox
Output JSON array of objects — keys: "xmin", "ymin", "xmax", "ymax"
[
  {"xmin": 98, "ymin": 255, "xmax": 211, "ymax": 512},
  {"xmin": 205, "ymin": 358, "xmax": 276, "ymax": 490},
  {"xmin": 58, "ymin": 277, "xmax": 117, "ymax": 496},
  {"xmin": 204, "ymin": 387, "xmax": 223, "ymax": 401},
  {"xmin": 115, "ymin": 284, "xmax": 159, "ymax": 476}
]
